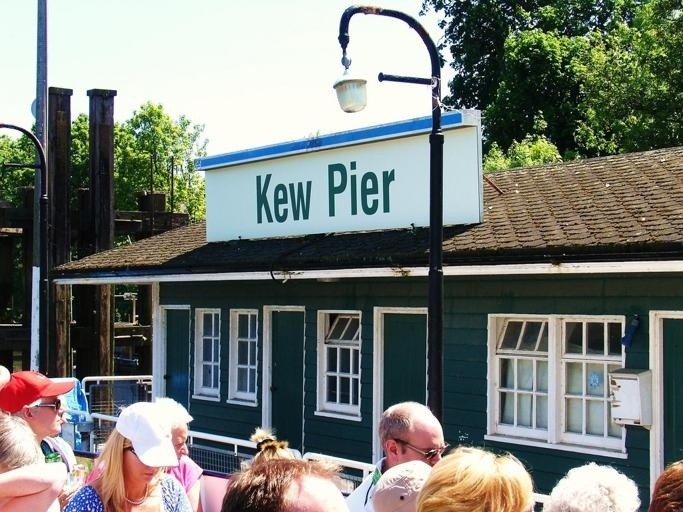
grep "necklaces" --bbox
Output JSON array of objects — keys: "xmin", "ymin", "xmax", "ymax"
[{"xmin": 120, "ymin": 480, "xmax": 150, "ymax": 506}]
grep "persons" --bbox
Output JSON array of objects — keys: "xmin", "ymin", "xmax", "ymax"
[
  {"xmin": 0, "ymin": 370, "xmax": 81, "ymax": 507},
  {"xmin": 0, "ymin": 413, "xmax": 67, "ymax": 512},
  {"xmin": 63, "ymin": 401, "xmax": 194, "ymax": 512},
  {"xmin": 155, "ymin": 396, "xmax": 201, "ymax": 512},
  {"xmin": 218, "ymin": 458, "xmax": 346, "ymax": 511},
  {"xmin": 347, "ymin": 401, "xmax": 445, "ymax": 511},
  {"xmin": 247, "ymin": 428, "xmax": 301, "ymax": 462},
  {"xmin": 650, "ymin": 458, "xmax": 683, "ymax": 511},
  {"xmin": 417, "ymin": 446, "xmax": 534, "ymax": 512},
  {"xmin": 543, "ymin": 463, "xmax": 642, "ymax": 512}
]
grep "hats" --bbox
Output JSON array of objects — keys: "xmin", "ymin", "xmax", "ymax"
[
  {"xmin": 115, "ymin": 401, "xmax": 180, "ymax": 468},
  {"xmin": 0, "ymin": 370, "xmax": 77, "ymax": 414}
]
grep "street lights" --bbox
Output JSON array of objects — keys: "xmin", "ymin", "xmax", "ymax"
[{"xmin": 334, "ymin": 4, "xmax": 443, "ymax": 426}]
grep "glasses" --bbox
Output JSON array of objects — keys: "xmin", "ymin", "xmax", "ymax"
[
  {"xmin": 35, "ymin": 399, "xmax": 62, "ymax": 411},
  {"xmin": 394, "ymin": 438, "xmax": 451, "ymax": 460},
  {"xmin": 127, "ymin": 446, "xmax": 135, "ymax": 454}
]
